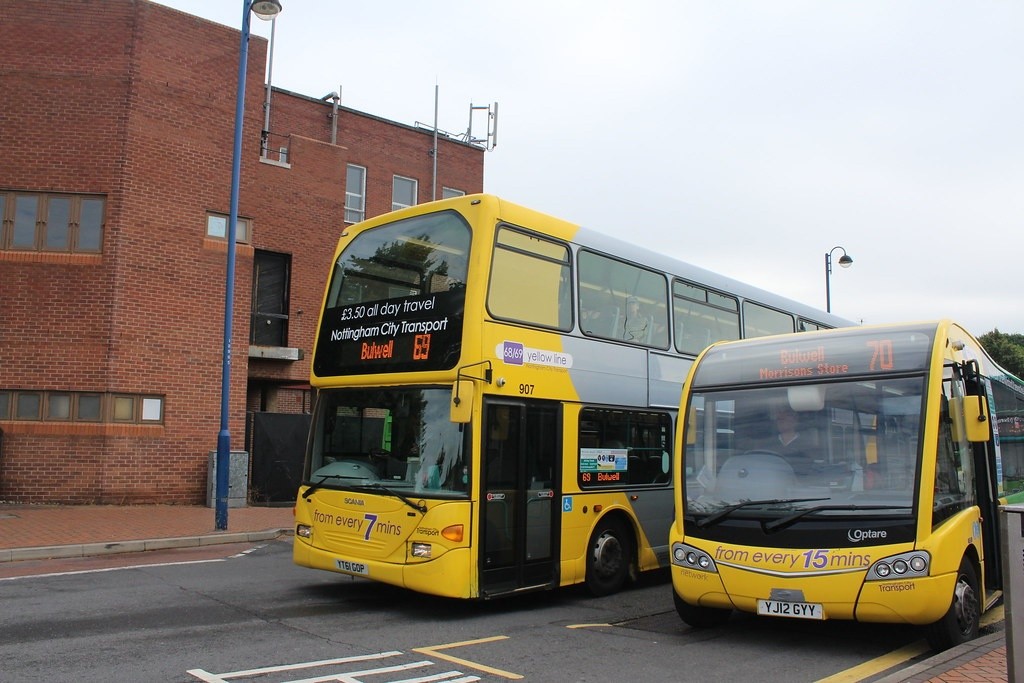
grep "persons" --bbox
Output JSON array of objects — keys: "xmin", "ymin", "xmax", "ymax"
[
  {"xmin": 620, "ymin": 297, "xmax": 647, "ymax": 343},
  {"xmin": 755, "ymin": 403, "xmax": 816, "ymax": 475}
]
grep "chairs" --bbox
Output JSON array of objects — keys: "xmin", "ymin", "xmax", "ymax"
[{"xmin": 601, "ymin": 439, "xmax": 669, "ymax": 484}]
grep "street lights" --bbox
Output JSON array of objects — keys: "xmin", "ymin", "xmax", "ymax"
[
  {"xmin": 213, "ymin": 0, "xmax": 281, "ymax": 531},
  {"xmin": 825, "ymin": 246, "xmax": 853, "ymax": 314}
]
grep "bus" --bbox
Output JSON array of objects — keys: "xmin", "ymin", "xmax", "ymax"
[
  {"xmin": 294, "ymin": 193, "xmax": 901, "ymax": 600},
  {"xmin": 668, "ymin": 320, "xmax": 1024, "ymax": 649}
]
